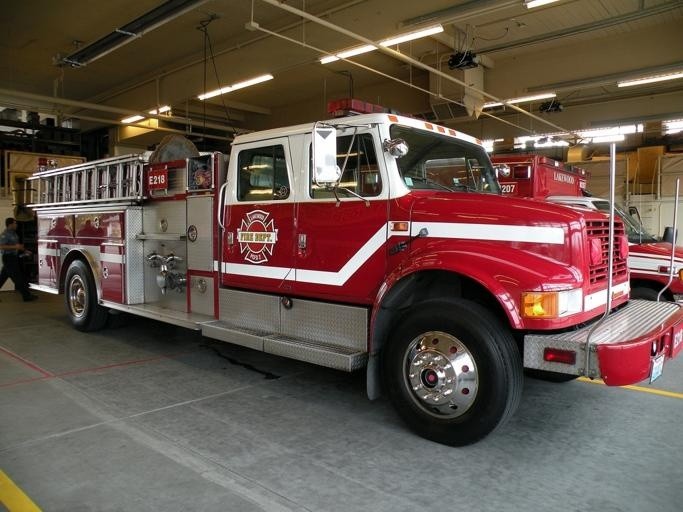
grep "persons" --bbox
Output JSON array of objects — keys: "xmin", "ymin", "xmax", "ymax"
[
  {"xmin": 75, "ymin": 217, "xmax": 100, "ymax": 246},
  {"xmin": 0, "ymin": 217, "xmax": 40, "ymax": 302},
  {"xmin": 45, "ymin": 218, "xmax": 74, "ymax": 276}
]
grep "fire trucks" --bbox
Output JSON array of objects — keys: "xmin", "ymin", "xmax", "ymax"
[
  {"xmin": 21, "ymin": 97, "xmax": 682, "ymax": 448},
  {"xmin": 425, "ymin": 152, "xmax": 682, "ymax": 303}
]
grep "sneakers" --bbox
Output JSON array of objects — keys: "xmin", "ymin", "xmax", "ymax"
[{"xmin": 23, "ymin": 295, "xmax": 37, "ymax": 302}]
